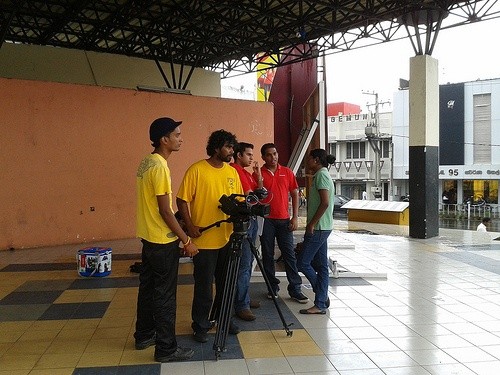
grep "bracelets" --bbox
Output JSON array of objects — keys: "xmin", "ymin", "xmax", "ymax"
[
  {"xmin": 257, "ymin": 177, "xmax": 264, "ymax": 182},
  {"xmin": 183, "ymin": 236, "xmax": 190, "ymax": 247},
  {"xmin": 310, "ymin": 221, "xmax": 316, "ymax": 225}
]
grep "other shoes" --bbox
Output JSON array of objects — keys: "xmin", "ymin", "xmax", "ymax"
[
  {"xmin": 300, "ymin": 305, "xmax": 326, "ymax": 314},
  {"xmin": 268, "ymin": 290, "xmax": 278, "ymax": 298},
  {"xmin": 134, "ymin": 334, "xmax": 156, "ymax": 350},
  {"xmin": 193, "ymin": 331, "xmax": 208, "ymax": 342},
  {"xmin": 153, "ymin": 345, "xmax": 194, "ymax": 361},
  {"xmin": 291, "ymin": 293, "xmax": 309, "ymax": 302},
  {"xmin": 238, "ymin": 308, "xmax": 256, "ymax": 320},
  {"xmin": 249, "ymin": 299, "xmax": 259, "ymax": 308},
  {"xmin": 326, "ymin": 298, "xmax": 330, "ymax": 308},
  {"xmin": 228, "ymin": 321, "xmax": 241, "ymax": 335}
]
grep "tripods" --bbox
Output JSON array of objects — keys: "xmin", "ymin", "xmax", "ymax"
[{"xmin": 199, "ymin": 219, "xmax": 293, "ymax": 362}]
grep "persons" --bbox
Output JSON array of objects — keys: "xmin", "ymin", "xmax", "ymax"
[
  {"xmin": 252, "ymin": 142, "xmax": 310, "ymax": 304},
  {"xmin": 133, "ymin": 117, "xmax": 201, "ymax": 363},
  {"xmin": 176, "ymin": 129, "xmax": 251, "ymax": 343},
  {"xmin": 298, "ymin": 148, "xmax": 337, "ymax": 315},
  {"xmin": 297, "ymin": 189, "xmax": 305, "ymax": 208},
  {"xmin": 476, "ymin": 217, "xmax": 491, "ymax": 232},
  {"xmin": 400, "ymin": 188, "xmax": 495, "ymax": 212},
  {"xmin": 173, "ymin": 210, "xmax": 191, "ymax": 258},
  {"xmin": 263, "ymin": 63, "xmax": 276, "ymax": 101},
  {"xmin": 229, "ymin": 141, "xmax": 264, "ymax": 321},
  {"xmin": 362, "ymin": 191, "xmax": 367, "ymax": 200},
  {"xmin": 375, "ymin": 188, "xmax": 380, "ymax": 193}
]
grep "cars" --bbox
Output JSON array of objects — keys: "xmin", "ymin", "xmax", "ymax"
[{"xmin": 333, "ymin": 195, "xmax": 351, "ymax": 214}]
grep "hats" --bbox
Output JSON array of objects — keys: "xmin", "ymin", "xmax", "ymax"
[{"xmin": 149, "ymin": 117, "xmax": 182, "ymax": 149}]
